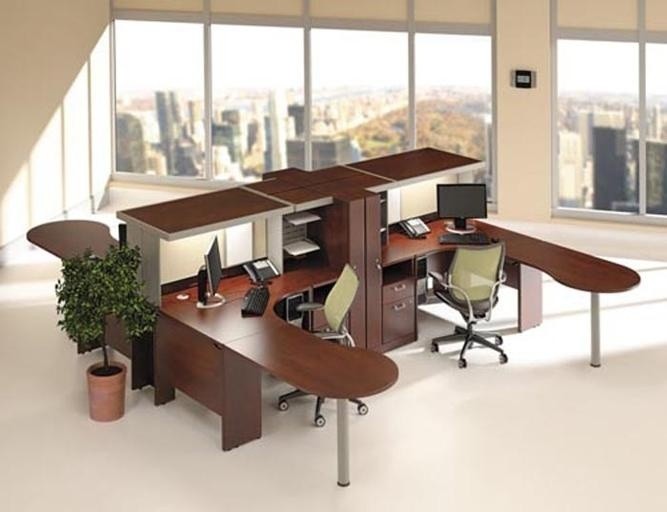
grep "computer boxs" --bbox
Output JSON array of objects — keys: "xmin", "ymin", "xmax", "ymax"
[
  {"xmin": 416, "ymin": 255, "xmax": 428, "ymax": 305},
  {"xmin": 277, "ymin": 292, "xmax": 304, "ymax": 328}
]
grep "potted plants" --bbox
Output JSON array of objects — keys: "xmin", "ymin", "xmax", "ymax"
[{"xmin": 55, "ymin": 243, "xmax": 160, "ymax": 422}]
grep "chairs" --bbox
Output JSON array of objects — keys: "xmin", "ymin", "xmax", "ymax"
[
  {"xmin": 277, "ymin": 264, "xmax": 369, "ymax": 426},
  {"xmin": 428, "ymin": 241, "xmax": 509, "ymax": 368}
]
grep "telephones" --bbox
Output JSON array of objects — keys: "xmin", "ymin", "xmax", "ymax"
[
  {"xmin": 242, "ymin": 257, "xmax": 281, "ymax": 283},
  {"xmin": 399, "ymin": 217, "xmax": 431, "ymax": 239}
]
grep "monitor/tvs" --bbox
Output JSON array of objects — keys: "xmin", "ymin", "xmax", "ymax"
[
  {"xmin": 436, "ymin": 183, "xmax": 488, "ymax": 235},
  {"xmin": 196, "ymin": 233, "xmax": 226, "ymax": 309}
]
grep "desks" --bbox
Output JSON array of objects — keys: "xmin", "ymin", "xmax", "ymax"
[
  {"xmin": 24, "ymin": 222, "xmax": 121, "ymax": 266},
  {"xmin": 114, "ymin": 170, "xmax": 398, "ymax": 486},
  {"xmin": 281, "ymin": 147, "xmax": 642, "ymax": 368}
]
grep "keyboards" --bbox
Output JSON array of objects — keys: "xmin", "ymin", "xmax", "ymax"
[
  {"xmin": 439, "ymin": 233, "xmax": 490, "ymax": 245},
  {"xmin": 240, "ymin": 286, "xmax": 269, "ymax": 316}
]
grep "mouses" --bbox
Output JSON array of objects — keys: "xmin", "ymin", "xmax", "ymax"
[{"xmin": 492, "ymin": 236, "xmax": 499, "ymax": 243}]
